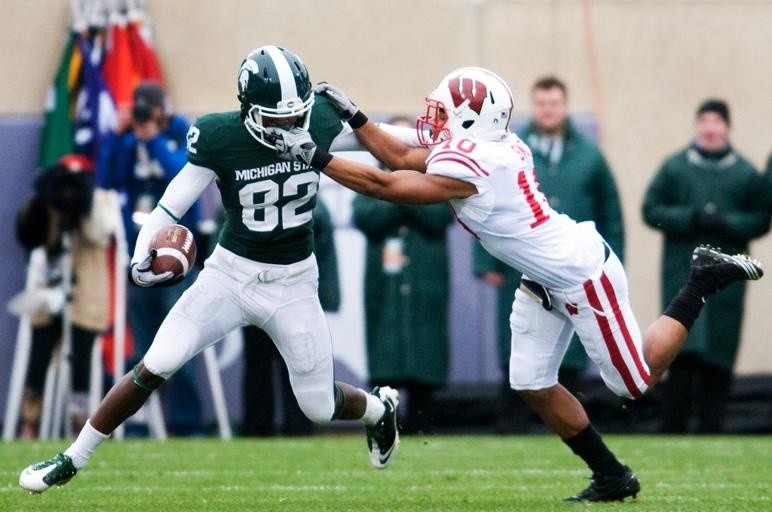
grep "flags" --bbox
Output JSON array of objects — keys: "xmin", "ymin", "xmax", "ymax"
[{"xmin": 29, "ymin": 12, "xmax": 169, "ymax": 190}]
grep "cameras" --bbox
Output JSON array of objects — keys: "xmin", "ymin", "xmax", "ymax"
[{"xmin": 133, "ymin": 103, "xmax": 152, "ymax": 121}]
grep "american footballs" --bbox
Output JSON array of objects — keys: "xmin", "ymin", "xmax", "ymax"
[{"xmin": 148, "ymin": 224, "xmax": 197, "ymax": 281}]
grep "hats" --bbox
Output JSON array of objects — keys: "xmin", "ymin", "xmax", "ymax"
[
  {"xmin": 697, "ymin": 100, "xmax": 729, "ymax": 124},
  {"xmin": 131, "ymin": 80, "xmax": 164, "ymax": 107}
]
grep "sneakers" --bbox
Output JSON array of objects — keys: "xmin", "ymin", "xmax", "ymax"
[
  {"xmin": 690, "ymin": 244, "xmax": 765, "ymax": 282},
  {"xmin": 18, "ymin": 453, "xmax": 77, "ymax": 497},
  {"xmin": 564, "ymin": 465, "xmax": 642, "ymax": 503},
  {"xmin": 363, "ymin": 386, "xmax": 401, "ymax": 470}
]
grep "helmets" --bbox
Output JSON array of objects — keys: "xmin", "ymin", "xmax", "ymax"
[
  {"xmin": 416, "ymin": 66, "xmax": 514, "ymax": 148},
  {"xmin": 237, "ymin": 45, "xmax": 313, "ymax": 152}
]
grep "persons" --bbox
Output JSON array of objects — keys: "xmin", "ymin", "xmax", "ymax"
[
  {"xmin": 470, "ymin": 75, "xmax": 626, "ymax": 434},
  {"xmin": 16, "ymin": 44, "xmax": 436, "ymax": 489},
  {"xmin": 637, "ymin": 95, "xmax": 772, "ymax": 434},
  {"xmin": 350, "ymin": 110, "xmax": 452, "ymax": 434},
  {"xmin": 211, "ymin": 187, "xmax": 342, "ymax": 440},
  {"xmin": 104, "ymin": 80, "xmax": 205, "ymax": 435},
  {"xmin": 262, "ymin": 64, "xmax": 765, "ymax": 503}
]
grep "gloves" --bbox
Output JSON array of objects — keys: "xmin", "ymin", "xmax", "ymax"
[
  {"xmin": 312, "ymin": 81, "xmax": 359, "ymax": 123},
  {"xmin": 128, "ymin": 249, "xmax": 184, "ymax": 287},
  {"xmin": 263, "ymin": 124, "xmax": 316, "ymax": 167}
]
